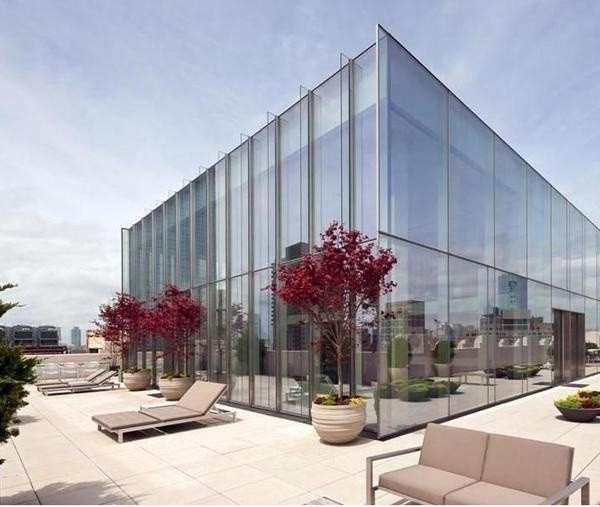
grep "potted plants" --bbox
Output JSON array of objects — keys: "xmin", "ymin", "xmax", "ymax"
[
  {"xmin": 554, "ymin": 389, "xmax": 600, "ymax": 421},
  {"xmin": 86, "ymin": 291, "xmax": 150, "ymax": 390},
  {"xmin": 386, "ymin": 336, "xmax": 416, "ymax": 381},
  {"xmin": 274, "ymin": 220, "xmax": 398, "ymax": 445},
  {"xmin": 150, "ymin": 283, "xmax": 208, "ymax": 400},
  {"xmin": 433, "ymin": 340, "xmax": 455, "ymax": 377}
]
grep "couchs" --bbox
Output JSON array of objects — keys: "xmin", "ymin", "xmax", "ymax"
[{"xmin": 366, "ymin": 422, "xmax": 590, "ymax": 505}]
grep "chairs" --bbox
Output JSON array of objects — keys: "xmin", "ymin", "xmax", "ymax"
[
  {"xmin": 37, "ymin": 370, "xmax": 118, "ymax": 396},
  {"xmin": 285, "ymin": 375, "xmax": 377, "ymax": 411},
  {"xmin": 92, "ymin": 380, "xmax": 237, "ymax": 443},
  {"xmin": 33, "ymin": 368, "xmax": 108, "ymax": 392}
]
